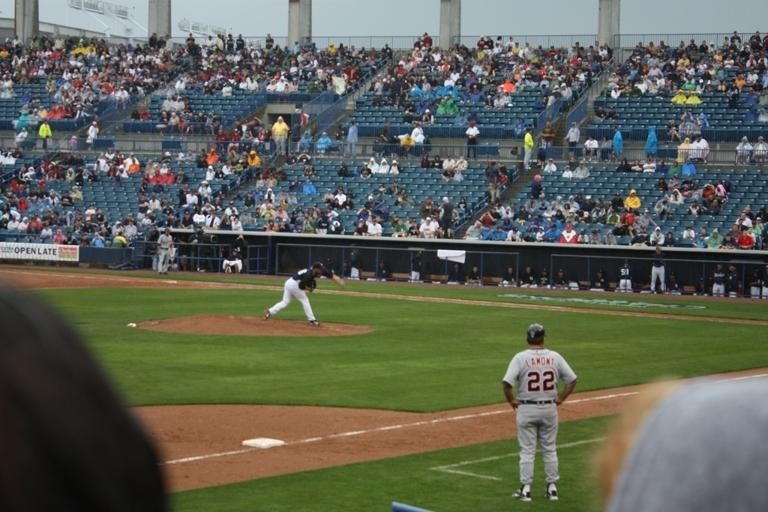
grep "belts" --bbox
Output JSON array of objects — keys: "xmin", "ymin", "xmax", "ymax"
[{"xmin": 522, "ymin": 400, "xmax": 555, "ymax": 405}]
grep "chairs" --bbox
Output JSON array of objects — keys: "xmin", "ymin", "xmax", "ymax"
[{"xmin": 1, "ymin": 74, "xmax": 768, "ymax": 241}]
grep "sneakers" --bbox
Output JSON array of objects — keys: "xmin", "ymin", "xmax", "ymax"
[
  {"xmin": 544, "ymin": 483, "xmax": 560, "ymax": 501},
  {"xmin": 512, "ymin": 484, "xmax": 532, "ymax": 502},
  {"xmin": 309, "ymin": 320, "xmax": 320, "ymax": 327},
  {"xmin": 262, "ymin": 306, "xmax": 270, "ymax": 321}
]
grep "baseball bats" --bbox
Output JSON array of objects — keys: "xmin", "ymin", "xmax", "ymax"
[{"xmin": 391, "ymin": 500, "xmax": 435, "ymax": 512}]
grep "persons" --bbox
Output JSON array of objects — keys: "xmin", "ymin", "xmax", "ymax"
[
  {"xmin": 501, "ymin": 323, "xmax": 576, "ymax": 502},
  {"xmin": 598, "ymin": 377, "xmax": 768, "ymax": 512},
  {"xmin": 0, "ymin": 280, "xmax": 177, "ymax": 512},
  {"xmin": 263, "ymin": 262, "xmax": 344, "ymax": 327}
]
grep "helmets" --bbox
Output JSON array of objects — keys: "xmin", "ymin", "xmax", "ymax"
[{"xmin": 527, "ymin": 323, "xmax": 545, "ymax": 339}]
